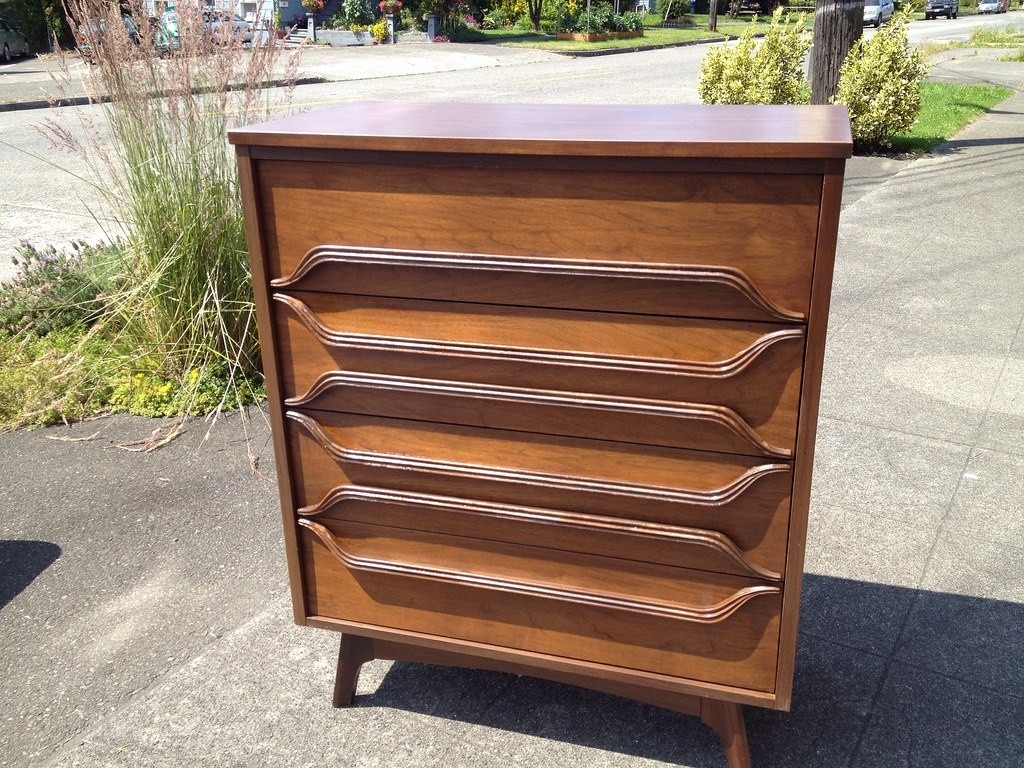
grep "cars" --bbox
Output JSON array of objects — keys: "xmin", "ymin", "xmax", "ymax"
[
  {"xmin": 863, "ymin": 0, "xmax": 894, "ymax": 28},
  {"xmin": 0, "ymin": 18, "xmax": 33, "ymax": 62},
  {"xmin": 76, "ymin": 5, "xmax": 254, "ymax": 56},
  {"xmin": 925, "ymin": 0, "xmax": 959, "ymax": 20},
  {"xmin": 977, "ymin": 0, "xmax": 1007, "ymax": 15}
]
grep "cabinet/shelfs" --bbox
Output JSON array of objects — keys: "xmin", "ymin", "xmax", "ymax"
[{"xmin": 227, "ymin": 101, "xmax": 852, "ymax": 768}]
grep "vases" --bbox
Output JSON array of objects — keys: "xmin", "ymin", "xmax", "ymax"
[
  {"xmin": 307, "ymin": 6, "xmax": 312, "ymax": 12},
  {"xmin": 387, "ymin": 7, "xmax": 396, "ymax": 13},
  {"xmin": 275, "ymin": 8, "xmax": 284, "ymax": 39}
]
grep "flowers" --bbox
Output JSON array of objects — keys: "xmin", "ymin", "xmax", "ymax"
[
  {"xmin": 301, "ymin": 0, "xmax": 324, "ymax": 13},
  {"xmin": 377, "ymin": 0, "xmax": 403, "ymax": 12}
]
